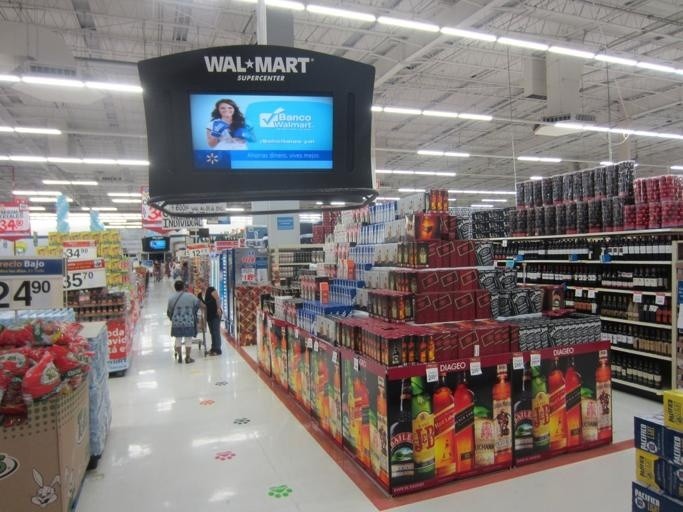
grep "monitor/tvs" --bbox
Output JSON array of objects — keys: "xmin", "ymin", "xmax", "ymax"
[
  {"xmin": 137, "ymin": 44, "xmax": 376, "ymax": 205},
  {"xmin": 142, "ymin": 235, "xmax": 170, "ymax": 251}
]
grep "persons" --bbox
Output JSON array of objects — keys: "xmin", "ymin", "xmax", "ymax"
[
  {"xmin": 167, "ymin": 281, "xmax": 200, "ymax": 364},
  {"xmin": 195, "ymin": 278, "xmax": 222, "ymax": 356},
  {"xmin": 152, "ymin": 242, "xmax": 157, "ymax": 249},
  {"xmin": 207, "ymin": 99, "xmax": 248, "ymax": 150},
  {"xmin": 154, "ymin": 257, "xmax": 189, "ymax": 285}
]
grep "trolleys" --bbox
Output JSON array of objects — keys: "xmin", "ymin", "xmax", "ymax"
[{"xmin": 172, "ymin": 312, "xmax": 209, "ymax": 360}]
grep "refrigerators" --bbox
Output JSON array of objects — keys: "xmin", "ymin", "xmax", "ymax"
[{"xmin": 221, "ymin": 248, "xmax": 254, "ymax": 340}]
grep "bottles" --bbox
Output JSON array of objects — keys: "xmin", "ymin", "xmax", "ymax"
[
  {"xmin": 428, "ymin": 189, "xmax": 447, "ymax": 213},
  {"xmin": 421, "ymin": 217, "xmax": 431, "ymax": 239},
  {"xmin": 308, "ymin": 345, "xmax": 341, "ymax": 448},
  {"xmin": 389, "ymin": 271, "xmax": 416, "ymax": 292},
  {"xmin": 338, "ymin": 358, "xmax": 388, "ymax": 489},
  {"xmin": 256, "ymin": 318, "xmax": 309, "ymax": 417},
  {"xmin": 367, "ymin": 290, "xmax": 413, "ymax": 322},
  {"xmin": 335, "ymin": 323, "xmax": 434, "ymax": 365},
  {"xmin": 511, "ymin": 355, "xmax": 611, "ymax": 459},
  {"xmin": 490, "ymin": 236, "xmax": 672, "ymax": 388},
  {"xmin": 389, "ymin": 368, "xmax": 513, "ymax": 485},
  {"xmin": 396, "ymin": 241, "xmax": 427, "ymax": 268}
]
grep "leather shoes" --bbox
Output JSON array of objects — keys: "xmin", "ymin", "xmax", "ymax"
[{"xmin": 210, "ymin": 352, "xmax": 221, "ymax": 356}]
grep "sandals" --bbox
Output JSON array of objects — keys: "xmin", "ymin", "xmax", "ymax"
[{"xmin": 186, "ymin": 358, "xmax": 195, "ymax": 363}]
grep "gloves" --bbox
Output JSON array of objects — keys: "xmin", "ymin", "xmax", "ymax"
[
  {"xmin": 234, "ymin": 128, "xmax": 251, "ymax": 141},
  {"xmin": 209, "ymin": 120, "xmax": 229, "ymax": 137}
]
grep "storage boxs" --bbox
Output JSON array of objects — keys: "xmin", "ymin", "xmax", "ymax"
[{"xmin": 629, "ymin": 389, "xmax": 682, "ymax": 511}]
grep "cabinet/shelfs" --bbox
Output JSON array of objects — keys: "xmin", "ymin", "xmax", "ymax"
[
  {"xmin": 486, "ymin": 229, "xmax": 672, "ymax": 397},
  {"xmin": 270, "ymin": 242, "xmax": 319, "ymax": 285}
]
